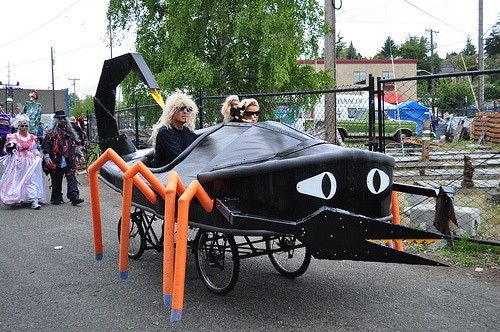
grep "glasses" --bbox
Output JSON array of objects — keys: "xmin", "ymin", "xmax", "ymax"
[
  {"xmin": 176, "ymin": 107, "xmax": 193, "ymax": 112},
  {"xmin": 244, "ymin": 111, "xmax": 261, "ymax": 117}
]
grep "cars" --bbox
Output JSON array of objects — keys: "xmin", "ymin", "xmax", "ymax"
[{"xmin": 272, "ymin": 106, "xmax": 296, "ymax": 125}]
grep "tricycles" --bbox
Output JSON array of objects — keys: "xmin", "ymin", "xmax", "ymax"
[{"xmin": 88, "ymin": 52, "xmax": 458, "ymax": 323}]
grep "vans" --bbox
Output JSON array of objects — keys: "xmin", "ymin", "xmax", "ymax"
[{"xmin": 310, "ymin": 95, "xmax": 399, "ymax": 121}]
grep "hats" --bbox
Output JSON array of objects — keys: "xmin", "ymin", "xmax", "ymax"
[{"xmin": 53, "ymin": 111, "xmax": 67, "ymax": 118}]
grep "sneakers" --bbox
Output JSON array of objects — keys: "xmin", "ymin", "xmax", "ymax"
[
  {"xmin": 31, "ymin": 202, "xmax": 41, "ymax": 209},
  {"xmin": 15, "ymin": 203, "xmax": 21, "ymax": 207}
]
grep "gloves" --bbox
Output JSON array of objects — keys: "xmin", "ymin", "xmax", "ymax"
[
  {"xmin": 12, "ymin": 149, "xmax": 17, "ymax": 154},
  {"xmin": 43, "ymin": 154, "xmax": 52, "ymax": 165}
]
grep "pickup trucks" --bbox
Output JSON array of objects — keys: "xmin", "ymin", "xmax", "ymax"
[{"xmin": 305, "ymin": 107, "xmax": 416, "ymax": 142}]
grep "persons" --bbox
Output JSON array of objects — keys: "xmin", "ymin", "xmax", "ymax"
[
  {"xmin": 23, "ymin": 89, "xmax": 42, "ymax": 135},
  {"xmin": 69, "ymin": 115, "xmax": 87, "ymax": 164},
  {"xmin": 148, "ymin": 92, "xmax": 199, "ymax": 168},
  {"xmin": 0, "ymin": 114, "xmax": 46, "ymax": 209},
  {"xmin": 41, "ymin": 111, "xmax": 85, "ymax": 206},
  {"xmin": 220, "ymin": 95, "xmax": 246, "ymax": 123},
  {"xmin": 240, "ymin": 98, "xmax": 260, "ymax": 123},
  {"xmin": 37, "ymin": 122, "xmax": 44, "ymax": 146}
]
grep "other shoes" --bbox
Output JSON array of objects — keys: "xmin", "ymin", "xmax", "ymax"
[{"xmin": 72, "ymin": 199, "xmax": 84, "ymax": 205}]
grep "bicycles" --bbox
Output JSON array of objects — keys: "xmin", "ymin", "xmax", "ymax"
[{"xmin": 79, "ymin": 141, "xmax": 101, "ymax": 169}]
